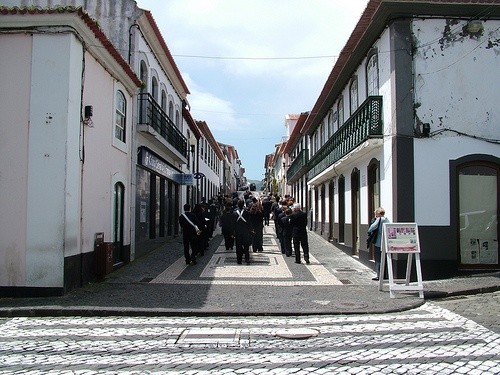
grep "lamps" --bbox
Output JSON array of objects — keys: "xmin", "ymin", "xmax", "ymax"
[{"xmin": 194, "ymin": 170, "xmax": 205, "ymax": 179}]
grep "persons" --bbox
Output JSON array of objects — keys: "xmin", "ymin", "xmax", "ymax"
[
  {"xmin": 179, "ymin": 204, "xmax": 199, "ymax": 264},
  {"xmin": 367, "ymin": 207, "xmax": 392, "ymax": 280},
  {"xmin": 371, "ymin": 209, "xmax": 378, "ymax": 225},
  {"xmin": 289, "ymin": 203, "xmax": 310, "ymax": 264},
  {"xmin": 192, "ymin": 189, "xmax": 297, "ymax": 256},
  {"xmin": 231, "ymin": 200, "xmax": 254, "ymax": 265}
]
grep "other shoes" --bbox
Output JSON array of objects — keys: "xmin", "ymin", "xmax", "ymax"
[
  {"xmin": 304, "ymin": 257, "xmax": 310, "ymax": 264},
  {"xmin": 369, "ymin": 259, "xmax": 376, "ymax": 262},
  {"xmin": 294, "ymin": 261, "xmax": 301, "ymax": 264},
  {"xmin": 186, "ymin": 261, "xmax": 191, "ymax": 265},
  {"xmin": 191, "ymin": 258, "xmax": 197, "ymax": 264}
]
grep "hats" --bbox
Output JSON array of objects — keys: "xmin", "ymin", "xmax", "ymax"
[{"xmin": 294, "ymin": 203, "xmax": 300, "ymax": 209}]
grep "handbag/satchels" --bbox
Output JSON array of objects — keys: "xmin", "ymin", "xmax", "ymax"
[
  {"xmin": 194, "ymin": 225, "xmax": 201, "ymax": 235},
  {"xmin": 369, "ymin": 217, "xmax": 381, "ymax": 244}
]
